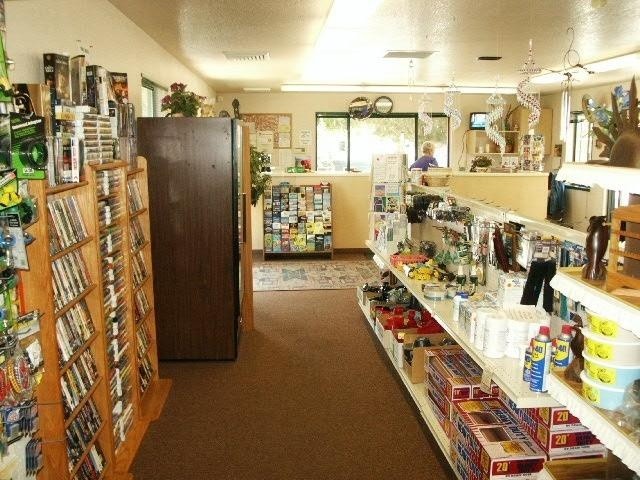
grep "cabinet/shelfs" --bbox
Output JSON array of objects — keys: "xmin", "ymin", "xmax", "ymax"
[
  {"xmin": 126, "ymin": 157, "xmax": 172, "ymax": 417},
  {"xmin": 262, "ymin": 183, "xmax": 333, "ymax": 260},
  {"xmin": 22, "ymin": 170, "xmax": 114, "ymax": 479},
  {"xmin": 356, "ymin": 183, "xmax": 620, "ymax": 479},
  {"xmin": 536, "ymin": 265, "xmax": 640, "ymax": 480},
  {"xmin": 511, "ymin": 107, "xmax": 553, "ymax": 155},
  {"xmin": 91, "ymin": 161, "xmax": 151, "ymax": 474},
  {"xmin": 136, "ymin": 116, "xmax": 254, "ymax": 363},
  {"xmin": 467, "ymin": 124, "xmax": 518, "ymax": 155}
]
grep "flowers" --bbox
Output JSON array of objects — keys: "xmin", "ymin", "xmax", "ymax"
[{"xmin": 159, "ymin": 81, "xmax": 202, "ymax": 114}]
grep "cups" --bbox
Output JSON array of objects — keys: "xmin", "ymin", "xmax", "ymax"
[{"xmin": 478, "ymin": 144, "xmax": 484, "ymax": 154}]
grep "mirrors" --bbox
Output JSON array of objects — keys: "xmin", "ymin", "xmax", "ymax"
[
  {"xmin": 346, "ymin": 95, "xmax": 374, "ymax": 119},
  {"xmin": 375, "ymin": 97, "xmax": 394, "ymax": 115},
  {"xmin": 1, "ymin": 166, "xmax": 60, "ymax": 479}
]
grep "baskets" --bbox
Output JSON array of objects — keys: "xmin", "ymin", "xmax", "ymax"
[
  {"xmin": 424, "ymin": 176, "xmax": 451, "ymax": 186},
  {"xmin": 390, "ymin": 254, "xmax": 426, "ymax": 267}
]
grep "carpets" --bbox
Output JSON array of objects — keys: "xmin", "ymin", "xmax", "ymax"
[{"xmin": 252, "ymin": 260, "xmax": 379, "ymax": 291}]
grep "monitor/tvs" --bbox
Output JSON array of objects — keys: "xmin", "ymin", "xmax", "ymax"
[{"xmin": 469, "ymin": 112, "xmax": 489, "ymax": 130}]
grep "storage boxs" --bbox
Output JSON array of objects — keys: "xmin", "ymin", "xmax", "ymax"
[{"xmin": 356, "ymin": 283, "xmax": 459, "ymax": 383}]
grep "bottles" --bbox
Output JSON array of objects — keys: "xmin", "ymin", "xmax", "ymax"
[
  {"xmin": 376, "ymin": 211, "xmax": 407, "ymax": 251},
  {"xmin": 485, "ymin": 144, "xmax": 489, "ymax": 153},
  {"xmin": 522, "ymin": 324, "xmax": 575, "ymax": 393}
]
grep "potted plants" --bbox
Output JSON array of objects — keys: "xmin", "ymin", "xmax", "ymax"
[{"xmin": 468, "ymin": 157, "xmax": 490, "ymax": 172}]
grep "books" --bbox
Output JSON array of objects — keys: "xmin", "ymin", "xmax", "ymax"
[
  {"xmin": 97, "ymin": 190, "xmax": 134, "ymax": 450},
  {"xmin": 43, "ymin": 53, "xmax": 138, "ymax": 189},
  {"xmin": 130, "ymin": 249, "xmax": 153, "ymax": 398},
  {"xmin": 60, "ymin": 347, "xmax": 99, "ymax": 423},
  {"xmin": 55, "ymin": 297, "xmax": 95, "ymax": 371},
  {"xmin": 47, "ymin": 194, "xmax": 89, "ymax": 257},
  {"xmin": 65, "ymin": 395, "xmax": 107, "ymax": 480},
  {"xmin": 128, "ymin": 179, "xmax": 145, "ymax": 254},
  {"xmin": 51, "ymin": 247, "xmax": 93, "ymax": 314}
]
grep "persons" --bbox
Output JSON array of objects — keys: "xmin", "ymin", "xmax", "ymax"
[{"xmin": 408, "ymin": 141, "xmax": 437, "ymax": 168}]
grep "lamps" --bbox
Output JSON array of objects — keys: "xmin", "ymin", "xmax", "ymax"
[
  {"xmin": 515, "ymin": 53, "xmax": 541, "ymax": 128},
  {"xmin": 484, "ymin": 93, "xmax": 514, "ymax": 162},
  {"xmin": 419, "ymin": 96, "xmax": 434, "ymax": 137},
  {"xmin": 443, "ymin": 75, "xmax": 463, "ymax": 130}
]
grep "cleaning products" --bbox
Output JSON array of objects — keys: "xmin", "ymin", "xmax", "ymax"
[
  {"xmin": 523, "ymin": 323, "xmax": 576, "ymax": 394},
  {"xmin": 458, "ymin": 299, "xmax": 546, "ymax": 359}
]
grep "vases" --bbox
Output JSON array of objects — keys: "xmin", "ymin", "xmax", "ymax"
[{"xmin": 172, "ymin": 108, "xmax": 201, "ymax": 117}]
